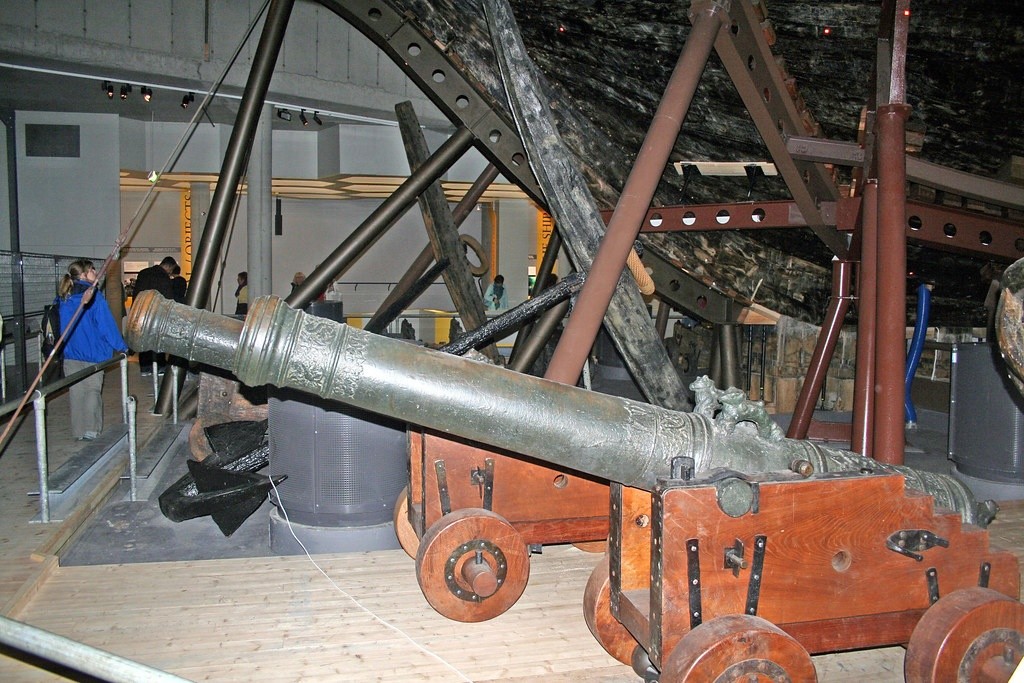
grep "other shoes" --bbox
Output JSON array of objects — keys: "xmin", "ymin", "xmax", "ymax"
[
  {"xmin": 141, "ymin": 367, "xmax": 153, "ymax": 376},
  {"xmin": 83, "ymin": 431, "xmax": 104, "ymax": 439},
  {"xmin": 157, "ymin": 364, "xmax": 169, "ymax": 376}
]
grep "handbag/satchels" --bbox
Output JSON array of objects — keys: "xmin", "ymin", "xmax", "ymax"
[{"xmin": 39, "ymin": 303, "xmax": 64, "ymax": 363}]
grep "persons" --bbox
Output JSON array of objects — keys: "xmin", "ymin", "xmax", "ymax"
[
  {"xmin": 121, "ymin": 256, "xmax": 340, "ymax": 374},
  {"xmin": 51, "ymin": 260, "xmax": 134, "ymax": 440},
  {"xmin": 482, "ymin": 274, "xmax": 558, "ymax": 310}
]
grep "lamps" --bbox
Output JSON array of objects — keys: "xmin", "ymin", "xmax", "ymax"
[
  {"xmin": 313, "ymin": 111, "xmax": 323, "ymax": 126},
  {"xmin": 140, "ymin": 86, "xmax": 153, "ymax": 102},
  {"xmin": 299, "ymin": 109, "xmax": 309, "ymax": 126},
  {"xmin": 101, "ymin": 82, "xmax": 114, "ymax": 98},
  {"xmin": 181, "ymin": 93, "xmax": 195, "ymax": 109},
  {"xmin": 120, "ymin": 84, "xmax": 133, "ymax": 100},
  {"xmin": 277, "ymin": 108, "xmax": 292, "ymax": 120}
]
